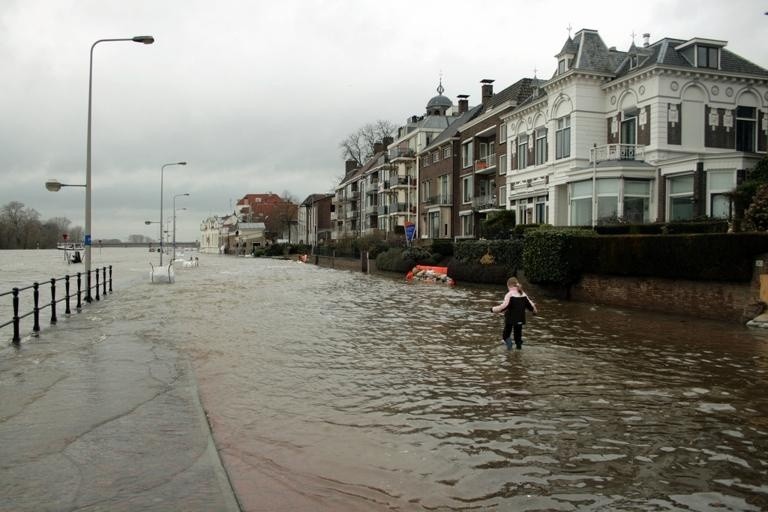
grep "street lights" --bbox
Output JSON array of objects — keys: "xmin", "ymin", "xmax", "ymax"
[
  {"xmin": 44, "ymin": 35, "xmax": 155, "ymax": 304},
  {"xmin": 145, "ymin": 159, "xmax": 190, "ymax": 268}
]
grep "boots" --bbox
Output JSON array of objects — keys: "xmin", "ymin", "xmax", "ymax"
[
  {"xmin": 504, "ymin": 336, "xmax": 513, "ymax": 350},
  {"xmin": 516, "ymin": 341, "xmax": 522, "ymax": 351}
]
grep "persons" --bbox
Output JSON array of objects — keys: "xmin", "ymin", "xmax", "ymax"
[{"xmin": 488, "ymin": 277, "xmax": 540, "ymax": 350}]
